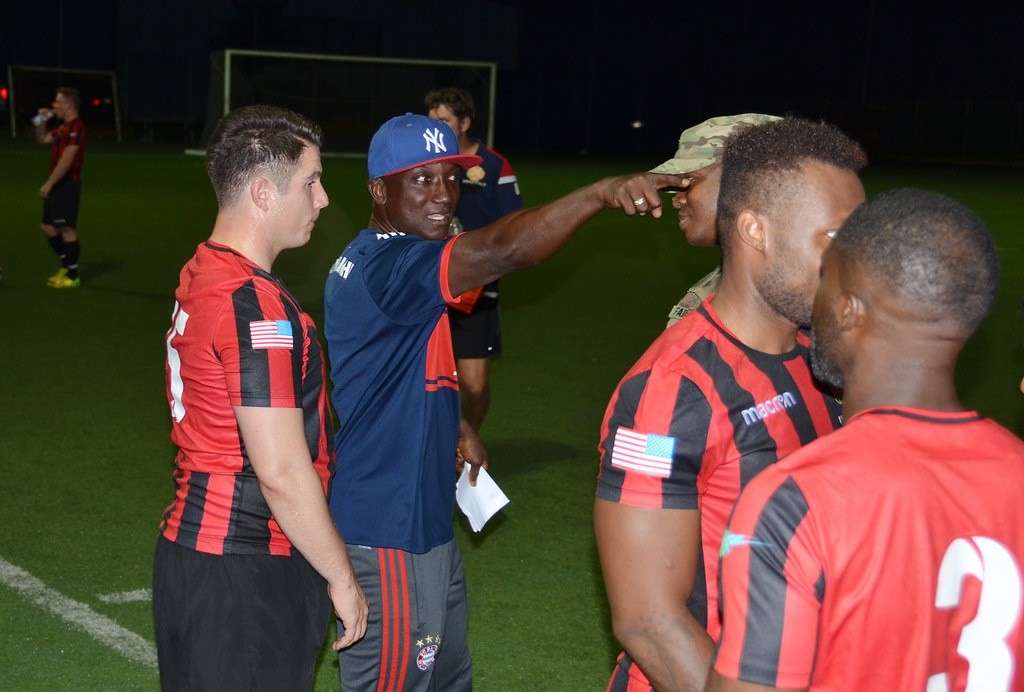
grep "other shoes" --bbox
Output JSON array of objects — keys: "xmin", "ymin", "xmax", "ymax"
[{"xmin": 47, "ymin": 268, "xmax": 81, "ymax": 287}]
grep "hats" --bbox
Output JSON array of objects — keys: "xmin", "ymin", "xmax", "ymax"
[
  {"xmin": 648, "ymin": 113, "xmax": 784, "ymax": 194},
  {"xmin": 367, "ymin": 112, "xmax": 484, "ymax": 181}
]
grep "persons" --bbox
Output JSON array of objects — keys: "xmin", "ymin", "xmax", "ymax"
[
  {"xmin": 32, "ymin": 90, "xmax": 88, "ymax": 288},
  {"xmin": 704, "ymin": 186, "xmax": 1024, "ymax": 692},
  {"xmin": 594, "ymin": 116, "xmax": 871, "ymax": 692},
  {"xmin": 423, "ymin": 87, "xmax": 523, "ymax": 433},
  {"xmin": 325, "ymin": 112, "xmax": 691, "ymax": 692},
  {"xmin": 152, "ymin": 106, "xmax": 370, "ymax": 692},
  {"xmin": 648, "ymin": 113, "xmax": 788, "ymax": 331}
]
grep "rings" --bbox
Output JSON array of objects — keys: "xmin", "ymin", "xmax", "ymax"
[{"xmin": 634, "ymin": 197, "xmax": 646, "ymax": 206}]
